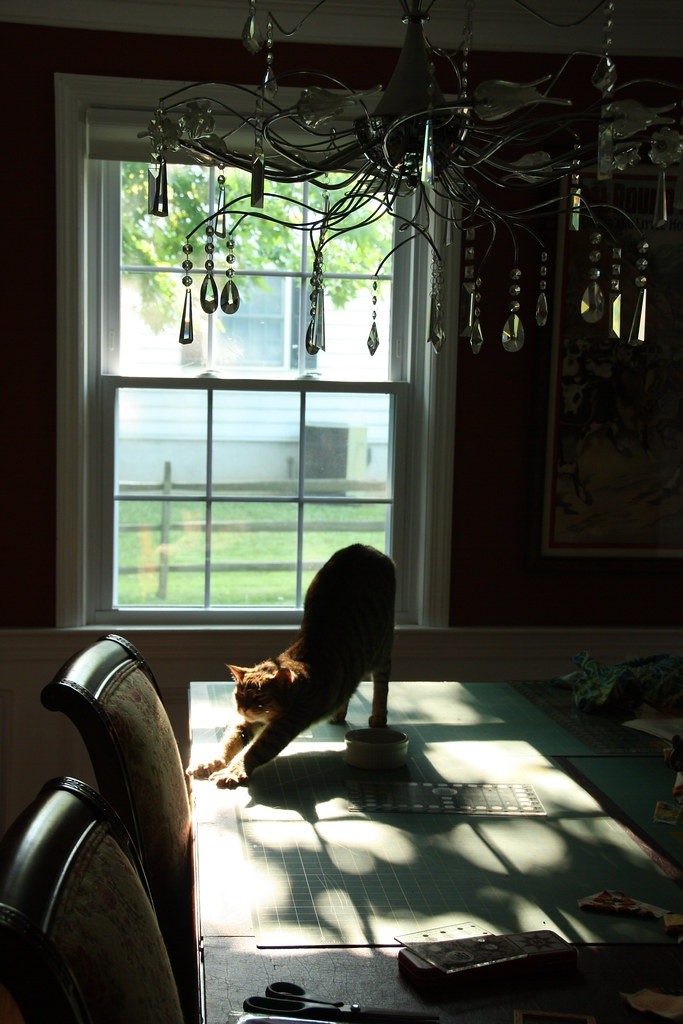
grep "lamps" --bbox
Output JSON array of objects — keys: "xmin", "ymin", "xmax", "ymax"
[{"xmin": 136, "ymin": 0, "xmax": 683, "ymax": 358}]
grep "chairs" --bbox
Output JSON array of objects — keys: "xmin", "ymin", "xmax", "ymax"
[
  {"xmin": 40, "ymin": 633, "xmax": 189, "ymax": 954},
  {"xmin": 1, "ymin": 775, "xmax": 194, "ymax": 1024}
]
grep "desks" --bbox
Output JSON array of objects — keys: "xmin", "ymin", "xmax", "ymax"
[{"xmin": 187, "ymin": 676, "xmax": 683, "ymax": 1024}]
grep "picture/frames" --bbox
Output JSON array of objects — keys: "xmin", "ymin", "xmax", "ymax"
[{"xmin": 520, "ymin": 142, "xmax": 683, "ymax": 575}]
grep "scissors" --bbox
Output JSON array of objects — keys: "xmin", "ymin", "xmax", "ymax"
[{"xmin": 243, "ymin": 981, "xmax": 440, "ymax": 1024}]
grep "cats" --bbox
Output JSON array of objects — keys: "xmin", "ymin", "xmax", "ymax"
[{"xmin": 186, "ymin": 542, "xmax": 397, "ymax": 791}]
